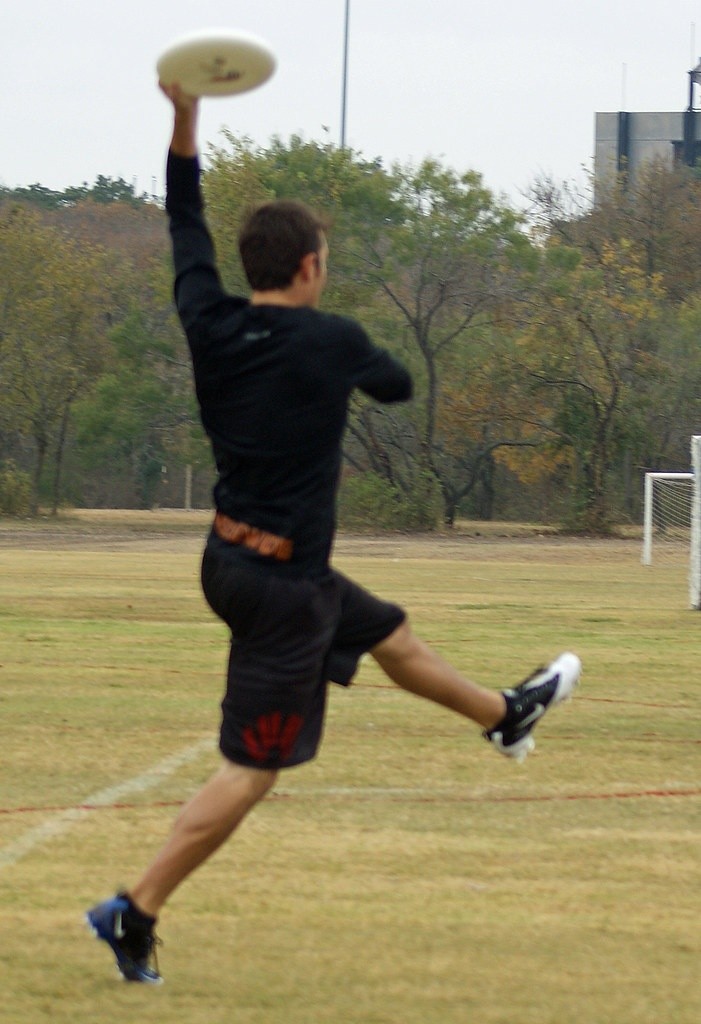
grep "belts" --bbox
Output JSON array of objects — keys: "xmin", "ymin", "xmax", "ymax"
[{"xmin": 215, "ymin": 515, "xmax": 293, "ymax": 559}]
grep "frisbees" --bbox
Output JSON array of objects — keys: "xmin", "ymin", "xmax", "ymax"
[{"xmin": 158, "ymin": 38, "xmax": 277, "ymax": 99}]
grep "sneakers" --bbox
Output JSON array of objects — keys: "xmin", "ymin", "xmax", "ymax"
[
  {"xmin": 83, "ymin": 894, "xmax": 163, "ymax": 983},
  {"xmin": 481, "ymin": 654, "xmax": 581, "ymax": 760}
]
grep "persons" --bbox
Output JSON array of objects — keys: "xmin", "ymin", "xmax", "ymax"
[{"xmin": 88, "ymin": 83, "xmax": 580, "ymax": 990}]
